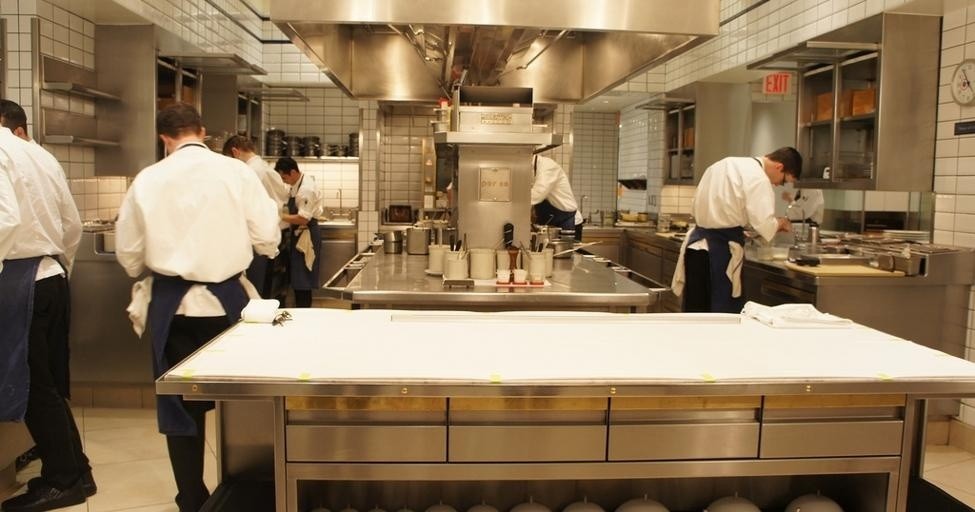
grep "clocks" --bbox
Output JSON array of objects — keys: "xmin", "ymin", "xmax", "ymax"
[{"xmin": 951, "ymin": 59, "xmax": 974, "ymax": 106}]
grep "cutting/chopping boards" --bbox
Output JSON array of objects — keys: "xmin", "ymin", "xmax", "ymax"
[{"xmin": 784, "ymin": 260, "xmax": 906, "ymax": 277}]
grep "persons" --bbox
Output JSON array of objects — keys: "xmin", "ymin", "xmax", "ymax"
[
  {"xmin": 532, "ymin": 153, "xmax": 583, "ymax": 241},
  {"xmin": 780, "ymin": 189, "xmax": 824, "ymax": 225},
  {"xmin": 671, "ymin": 146, "xmax": 802, "ymax": 312}
]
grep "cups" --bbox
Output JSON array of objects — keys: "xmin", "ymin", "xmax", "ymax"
[
  {"xmin": 808, "ymin": 221, "xmax": 819, "ymax": 244},
  {"xmin": 428, "ymin": 244, "xmax": 553, "ymax": 285}
]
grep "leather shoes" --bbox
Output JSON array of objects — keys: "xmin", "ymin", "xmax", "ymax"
[{"xmin": 1, "ymin": 469, "xmax": 97, "ymax": 512}]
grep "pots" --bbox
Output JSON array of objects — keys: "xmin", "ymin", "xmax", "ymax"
[
  {"xmin": 434, "ymin": 223, "xmax": 457, "ymax": 245},
  {"xmin": 374, "ymin": 231, "xmax": 403, "ymax": 255},
  {"xmin": 547, "ymin": 236, "xmax": 575, "ymax": 259},
  {"xmin": 265, "ymin": 128, "xmax": 360, "ymax": 157}
]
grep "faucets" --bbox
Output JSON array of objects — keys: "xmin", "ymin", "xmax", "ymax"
[
  {"xmin": 580, "ymin": 193, "xmax": 589, "ymax": 215},
  {"xmin": 785, "ymin": 202, "xmax": 805, "ymax": 240},
  {"xmin": 335, "ymin": 186, "xmax": 345, "ymax": 214}
]
